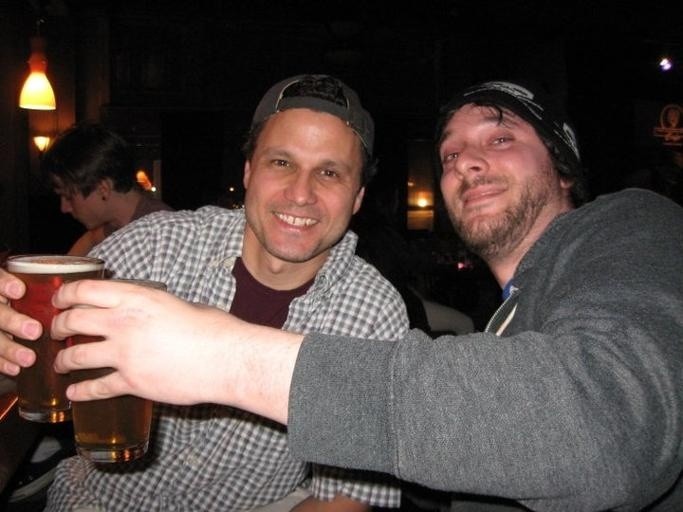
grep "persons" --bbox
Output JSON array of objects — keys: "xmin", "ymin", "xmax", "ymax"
[
  {"xmin": 47, "ymin": 78, "xmax": 682, "ymax": 511},
  {"xmin": 39, "ymin": 112, "xmax": 171, "ymax": 263},
  {"xmin": 0, "ymin": 74, "xmax": 412, "ymax": 510}
]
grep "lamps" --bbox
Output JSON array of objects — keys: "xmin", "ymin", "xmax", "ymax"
[{"xmin": 18, "ymin": 20, "xmax": 58, "ymax": 113}]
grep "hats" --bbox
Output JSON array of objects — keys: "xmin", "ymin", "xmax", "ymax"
[
  {"xmin": 248, "ymin": 73, "xmax": 376, "ymax": 159},
  {"xmin": 450, "ymin": 79, "xmax": 580, "ymax": 168}
]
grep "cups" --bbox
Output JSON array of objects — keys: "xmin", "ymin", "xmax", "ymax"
[
  {"xmin": 64, "ymin": 275, "xmax": 171, "ymax": 471},
  {"xmin": 7, "ymin": 253, "xmax": 107, "ymax": 426}
]
